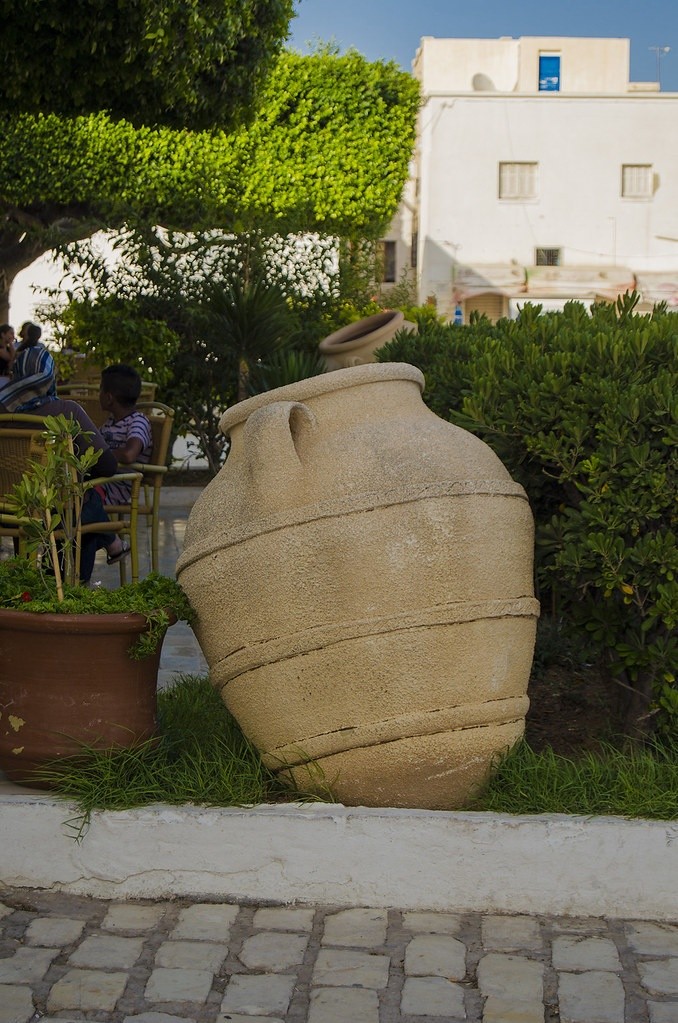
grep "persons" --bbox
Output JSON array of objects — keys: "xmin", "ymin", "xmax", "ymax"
[{"xmin": 0, "ymin": 321, "xmax": 155, "ymax": 586}]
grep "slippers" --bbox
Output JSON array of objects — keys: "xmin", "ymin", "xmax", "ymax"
[{"xmin": 107, "ymin": 540, "xmax": 131, "ymax": 564}]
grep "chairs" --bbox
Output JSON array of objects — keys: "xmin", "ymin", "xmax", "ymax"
[{"xmin": 0, "ymin": 382, "xmax": 174, "ymax": 587}]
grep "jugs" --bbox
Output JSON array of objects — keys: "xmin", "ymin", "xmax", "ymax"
[
  {"xmin": 316, "ymin": 310, "xmax": 418, "ymax": 373},
  {"xmin": 173, "ymin": 362, "xmax": 540, "ymax": 806}
]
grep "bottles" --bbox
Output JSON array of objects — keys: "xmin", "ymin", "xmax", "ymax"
[{"xmin": 41, "ymin": 431, "xmax": 56, "ymax": 455}]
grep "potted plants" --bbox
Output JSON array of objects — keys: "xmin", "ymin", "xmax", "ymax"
[{"xmin": 0, "ymin": 413, "xmax": 170, "ymax": 785}]
